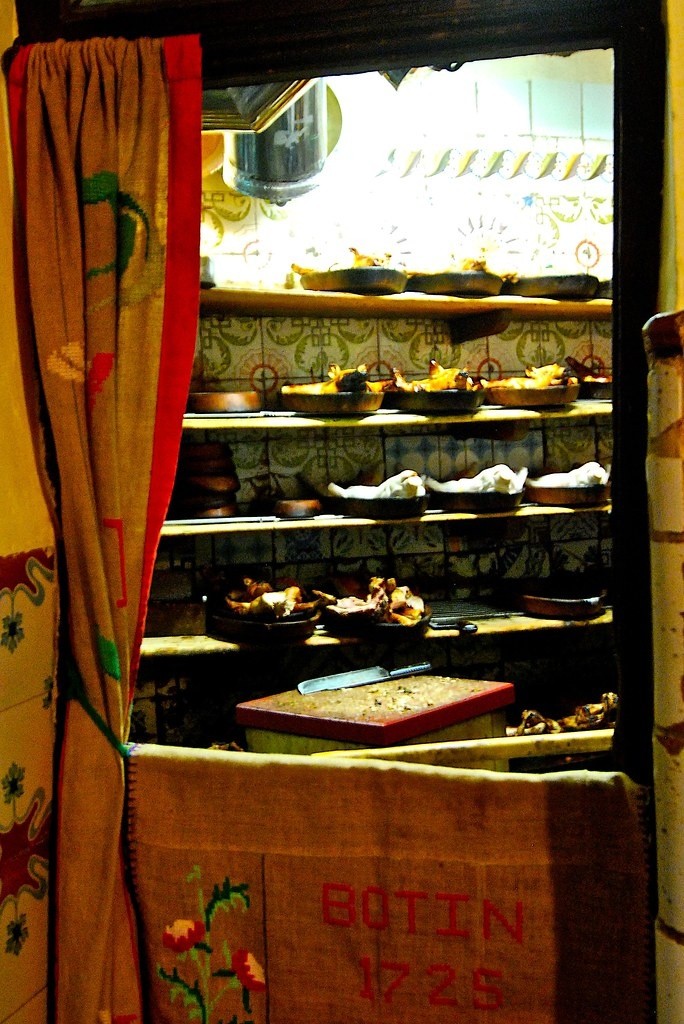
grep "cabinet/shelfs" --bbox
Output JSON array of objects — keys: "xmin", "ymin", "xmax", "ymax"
[{"xmin": 141, "ymin": 285, "xmax": 612, "ymax": 663}]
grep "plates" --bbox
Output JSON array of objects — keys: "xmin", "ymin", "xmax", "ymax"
[{"xmin": 196, "ymin": 256, "xmax": 610, "ymax": 641}]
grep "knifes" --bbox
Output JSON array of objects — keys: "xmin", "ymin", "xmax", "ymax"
[
  {"xmin": 429, "ymin": 618, "xmax": 478, "ymax": 634},
  {"xmin": 294, "ymin": 661, "xmax": 431, "ymax": 696}
]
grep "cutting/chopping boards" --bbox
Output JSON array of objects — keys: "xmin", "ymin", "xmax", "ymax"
[{"xmin": 235, "ymin": 674, "xmax": 515, "ymax": 746}]
